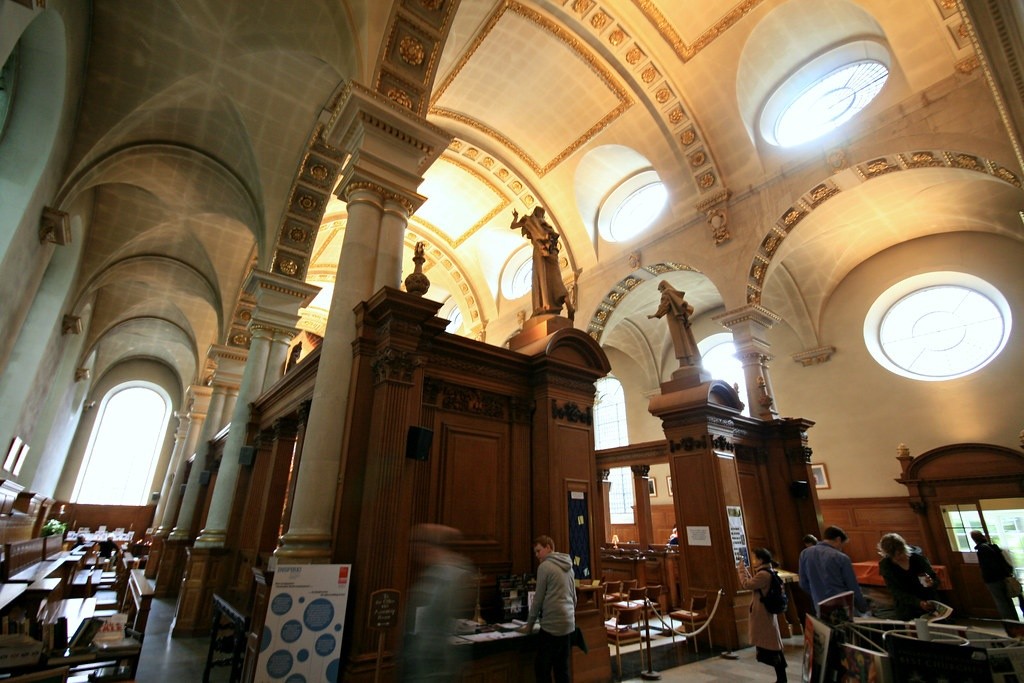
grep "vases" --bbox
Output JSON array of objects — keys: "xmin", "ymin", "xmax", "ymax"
[{"xmin": 405, "ymin": 257, "xmax": 430, "ymax": 297}]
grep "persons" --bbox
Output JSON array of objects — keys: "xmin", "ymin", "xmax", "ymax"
[
  {"xmin": 519, "ymin": 536, "xmax": 577, "ymax": 683},
  {"xmin": 743, "ymin": 547, "xmax": 791, "ymax": 683},
  {"xmin": 804, "ymin": 533, "xmax": 818, "ymax": 548},
  {"xmin": 878, "ymin": 532, "xmax": 941, "ymax": 618},
  {"xmin": 970, "ymin": 530, "xmax": 1024, "ymax": 623},
  {"xmin": 797, "ymin": 526, "xmax": 868, "ymax": 640},
  {"xmin": 669, "ymin": 526, "xmax": 678, "ymax": 545},
  {"xmin": 405, "ymin": 523, "xmax": 483, "ymax": 683}
]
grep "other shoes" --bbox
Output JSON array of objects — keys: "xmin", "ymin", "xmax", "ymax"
[{"xmin": 574, "ymin": 628, "xmax": 588, "ymax": 654}]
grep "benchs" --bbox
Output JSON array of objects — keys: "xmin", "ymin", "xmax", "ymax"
[
  {"xmin": 0, "ymin": 533, "xmax": 72, "ymax": 641},
  {"xmin": 600, "ymin": 543, "xmax": 680, "ymax": 612}
]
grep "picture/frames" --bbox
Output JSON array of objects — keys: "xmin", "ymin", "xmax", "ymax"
[
  {"xmin": 648, "ymin": 477, "xmax": 657, "ymax": 497},
  {"xmin": 666, "ymin": 476, "xmax": 673, "ymax": 496},
  {"xmin": 811, "ymin": 463, "xmax": 831, "ymax": 490}
]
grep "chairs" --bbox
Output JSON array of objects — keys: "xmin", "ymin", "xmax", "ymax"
[
  {"xmin": 73, "ymin": 545, "xmax": 155, "ymax": 683},
  {"xmin": 603, "ymin": 580, "xmax": 712, "ymax": 681}
]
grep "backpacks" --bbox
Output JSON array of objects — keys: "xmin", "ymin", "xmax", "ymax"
[{"xmin": 758, "ymin": 568, "xmax": 787, "ymax": 614}]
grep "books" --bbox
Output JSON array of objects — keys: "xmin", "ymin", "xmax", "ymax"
[{"xmin": 0, "ymin": 617, "xmax": 140, "ymax": 683}]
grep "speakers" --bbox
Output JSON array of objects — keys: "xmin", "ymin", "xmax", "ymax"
[
  {"xmin": 794, "ymin": 480, "xmax": 808, "ymax": 502},
  {"xmin": 180, "ymin": 483, "xmax": 186, "ymax": 496},
  {"xmin": 238, "ymin": 444, "xmax": 257, "ymax": 465},
  {"xmin": 405, "ymin": 425, "xmax": 434, "ymax": 461},
  {"xmin": 198, "ymin": 471, "xmax": 211, "ymax": 485},
  {"xmin": 151, "ymin": 492, "xmax": 160, "ymax": 501}
]
